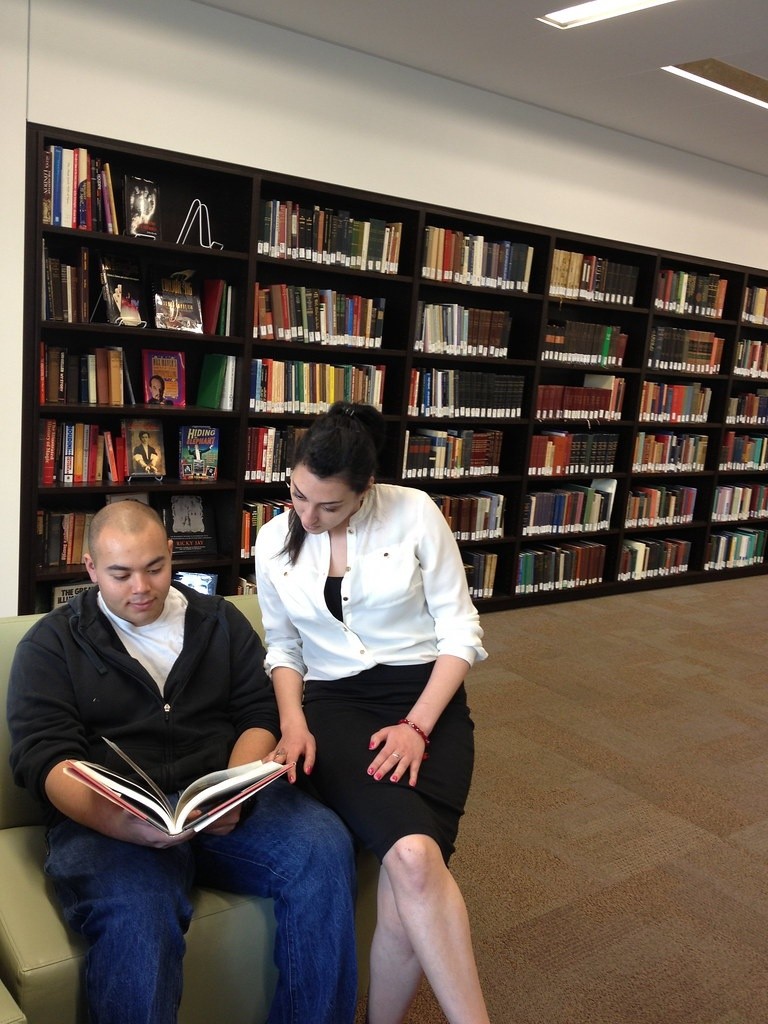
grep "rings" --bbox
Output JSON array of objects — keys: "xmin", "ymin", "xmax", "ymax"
[
  {"xmin": 275, "ymin": 752, "xmax": 286, "ymax": 757},
  {"xmin": 286, "ymin": 761, "xmax": 297, "ymax": 766},
  {"xmin": 392, "ymin": 754, "xmax": 401, "ymax": 760}
]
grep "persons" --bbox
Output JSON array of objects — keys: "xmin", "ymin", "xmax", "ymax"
[
  {"xmin": 6, "ymin": 498, "xmax": 362, "ymax": 1023},
  {"xmin": 255, "ymin": 401, "xmax": 494, "ymax": 1023}
]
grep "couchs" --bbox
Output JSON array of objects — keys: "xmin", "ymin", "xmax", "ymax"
[{"xmin": 3, "ymin": 595, "xmax": 277, "ymax": 1024}]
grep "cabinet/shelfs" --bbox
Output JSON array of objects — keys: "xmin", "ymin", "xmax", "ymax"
[
  {"xmin": 695, "ymin": 264, "xmax": 768, "ymax": 586},
  {"xmin": 612, "ymin": 245, "xmax": 747, "ymax": 591},
  {"xmin": 233, "ymin": 165, "xmax": 424, "ymax": 608},
  {"xmin": 509, "ymin": 225, "xmax": 659, "ymax": 608},
  {"xmin": 28, "ymin": 121, "xmax": 252, "ymax": 599},
  {"xmin": 399, "ymin": 200, "xmax": 554, "ymax": 610}
]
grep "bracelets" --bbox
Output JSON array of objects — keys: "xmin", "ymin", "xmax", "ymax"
[{"xmin": 398, "ymin": 718, "xmax": 430, "ymax": 745}]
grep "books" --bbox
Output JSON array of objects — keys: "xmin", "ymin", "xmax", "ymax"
[
  {"xmin": 37, "ymin": 142, "xmax": 768, "ymax": 614},
  {"xmin": 64, "ymin": 737, "xmax": 296, "ymax": 840}
]
